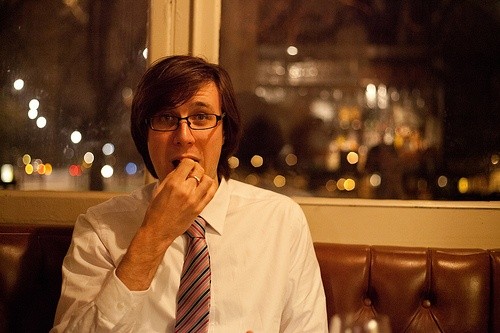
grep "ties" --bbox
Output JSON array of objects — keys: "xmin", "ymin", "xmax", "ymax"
[{"xmin": 173, "ymin": 215, "xmax": 212, "ymax": 333}]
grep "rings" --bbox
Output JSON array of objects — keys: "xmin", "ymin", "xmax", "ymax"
[{"xmin": 187, "ymin": 174, "xmax": 201, "ymax": 186}]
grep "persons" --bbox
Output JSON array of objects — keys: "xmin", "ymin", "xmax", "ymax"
[{"xmin": 50, "ymin": 56, "xmax": 331, "ymax": 333}]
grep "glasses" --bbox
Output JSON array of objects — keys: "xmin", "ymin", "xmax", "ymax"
[{"xmin": 141, "ymin": 109, "xmax": 227, "ymax": 133}]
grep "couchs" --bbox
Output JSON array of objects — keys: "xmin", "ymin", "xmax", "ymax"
[{"xmin": 314, "ymin": 240, "xmax": 500, "ymax": 333}]
